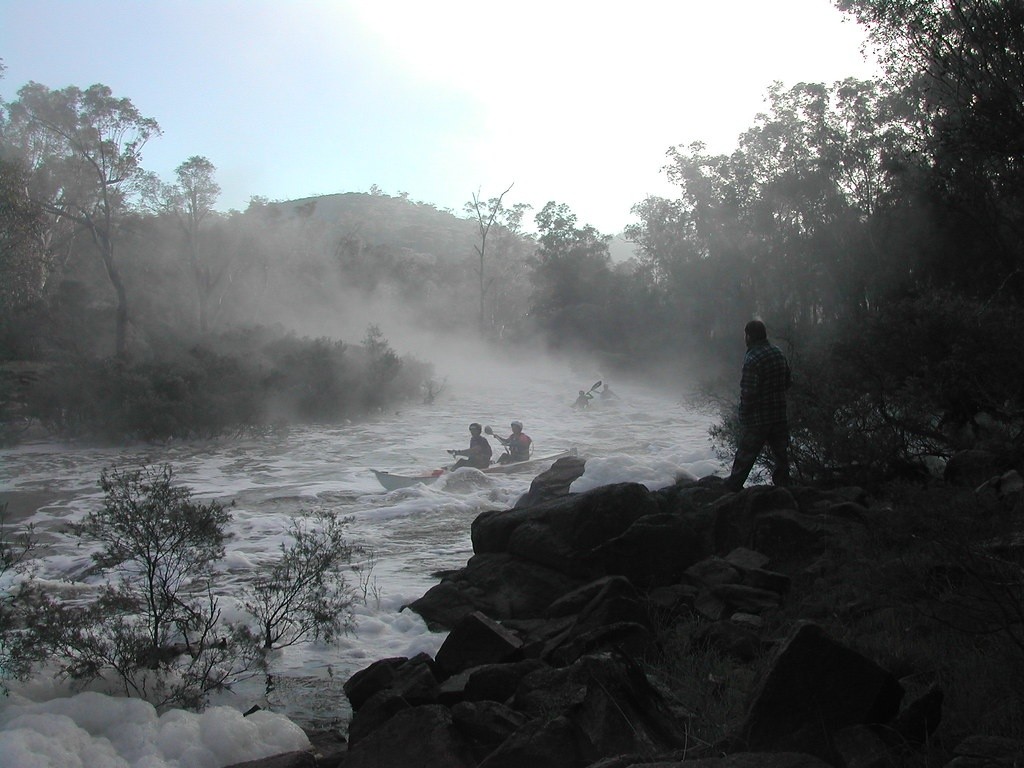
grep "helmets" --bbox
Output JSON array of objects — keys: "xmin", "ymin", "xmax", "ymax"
[{"xmin": 511, "ymin": 420, "xmax": 523, "ymax": 430}]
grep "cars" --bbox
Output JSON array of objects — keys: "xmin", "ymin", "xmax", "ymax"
[{"xmin": 371, "ymin": 447, "xmax": 579, "ymax": 490}]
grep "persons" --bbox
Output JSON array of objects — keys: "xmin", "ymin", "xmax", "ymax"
[
  {"xmin": 495, "ymin": 421, "xmax": 532, "ymax": 464},
  {"xmin": 725, "ymin": 321, "xmax": 795, "ymax": 492},
  {"xmin": 577, "ymin": 382, "xmax": 619, "ymax": 407},
  {"xmin": 448, "ymin": 424, "xmax": 492, "ymax": 472}
]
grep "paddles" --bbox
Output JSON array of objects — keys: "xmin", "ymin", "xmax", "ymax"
[
  {"xmin": 484, "ymin": 425, "xmax": 510, "ymax": 454},
  {"xmin": 585, "ymin": 381, "xmax": 602, "ymax": 396}
]
